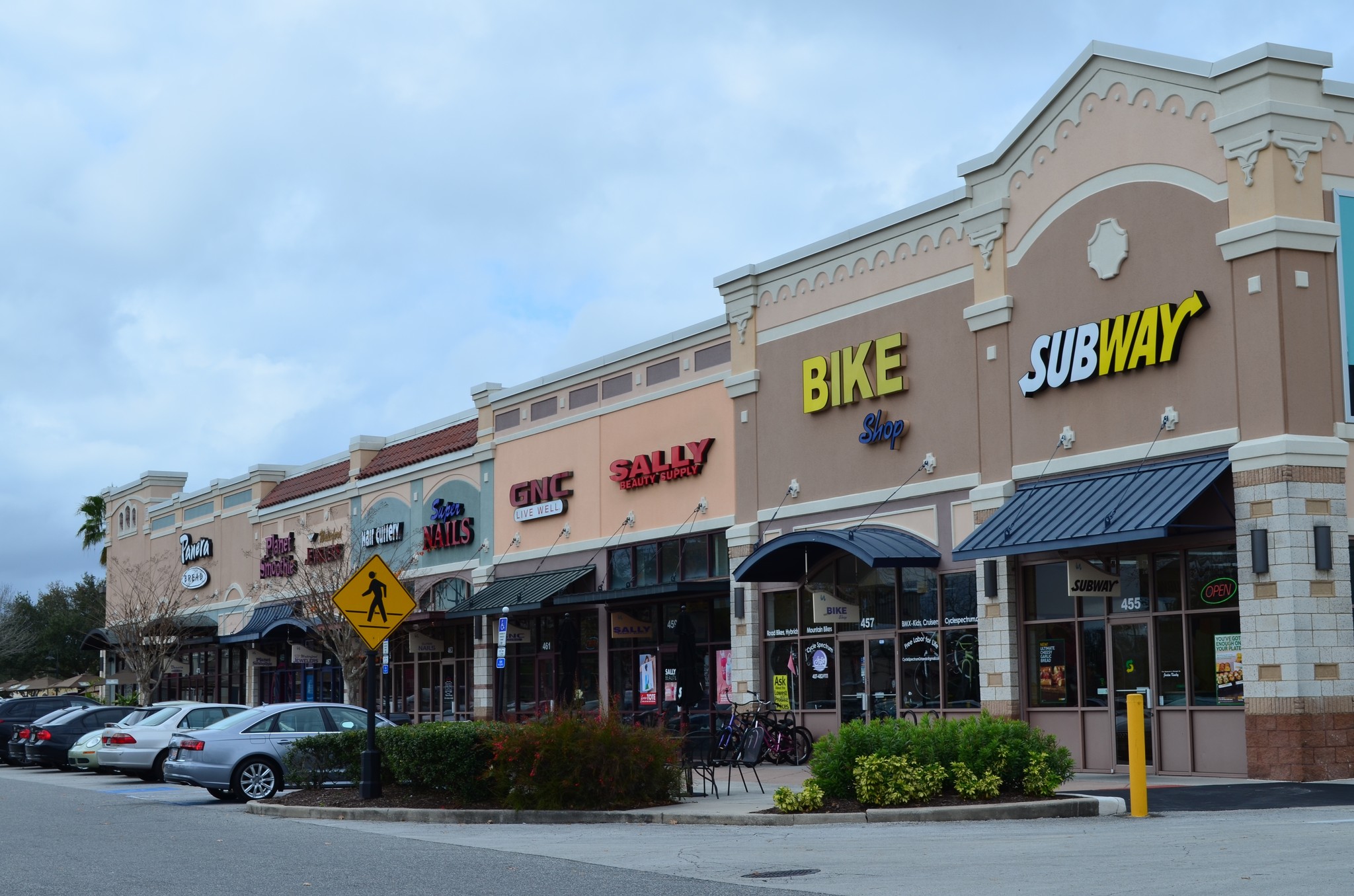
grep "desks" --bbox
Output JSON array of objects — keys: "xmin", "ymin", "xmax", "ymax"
[{"xmin": 659, "ymin": 735, "xmax": 717, "ymax": 797}]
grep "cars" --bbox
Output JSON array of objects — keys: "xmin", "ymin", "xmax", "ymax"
[
  {"xmin": 1, "ymin": 693, "xmax": 408, "ymax": 803},
  {"xmin": 797, "ymin": 692, "xmax": 1247, "ymax": 759},
  {"xmin": 375, "ymin": 698, "xmax": 728, "ymax": 755}
]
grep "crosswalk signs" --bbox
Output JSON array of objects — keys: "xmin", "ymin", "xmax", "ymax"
[{"xmin": 331, "ymin": 553, "xmax": 417, "ymax": 651}]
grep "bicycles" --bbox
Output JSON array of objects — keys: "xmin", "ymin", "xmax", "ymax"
[{"xmin": 717, "ymin": 689, "xmax": 817, "ymax": 767}]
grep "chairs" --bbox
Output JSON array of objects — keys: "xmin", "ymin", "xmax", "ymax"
[
  {"xmin": 665, "ymin": 731, "xmax": 719, "ymax": 801},
  {"xmin": 663, "ymin": 729, "xmax": 688, "ymax": 794},
  {"xmin": 710, "ymin": 727, "xmax": 765, "ymax": 796}
]
